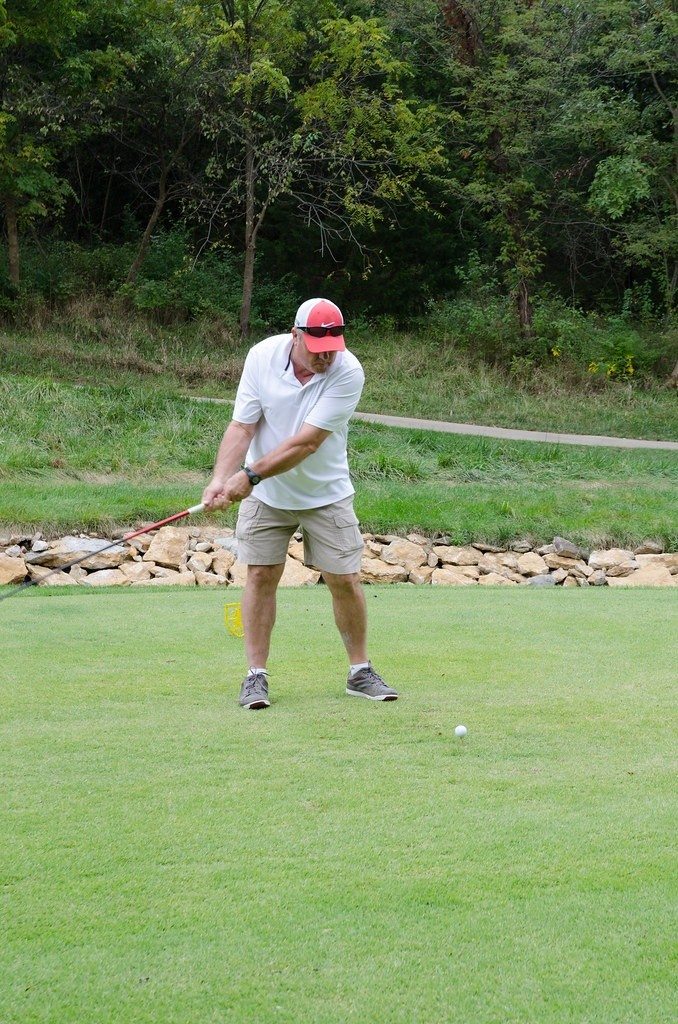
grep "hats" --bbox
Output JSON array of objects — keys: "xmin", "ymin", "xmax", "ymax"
[{"xmin": 294, "ymin": 298, "xmax": 346, "ymax": 353}]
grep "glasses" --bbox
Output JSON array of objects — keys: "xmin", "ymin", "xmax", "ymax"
[{"xmin": 296, "ymin": 325, "xmax": 346, "ymax": 338}]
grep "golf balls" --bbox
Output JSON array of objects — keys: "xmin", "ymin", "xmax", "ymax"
[{"xmin": 455, "ymin": 725, "xmax": 467, "ymax": 737}]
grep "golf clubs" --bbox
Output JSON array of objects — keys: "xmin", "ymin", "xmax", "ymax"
[{"xmin": 1, "ymin": 503, "xmax": 206, "ymax": 598}]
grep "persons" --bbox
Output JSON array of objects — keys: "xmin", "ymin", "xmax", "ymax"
[{"xmin": 202, "ymin": 298, "xmax": 398, "ymax": 710}]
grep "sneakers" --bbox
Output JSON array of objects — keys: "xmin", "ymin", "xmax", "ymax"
[
  {"xmin": 239, "ymin": 665, "xmax": 270, "ymax": 709},
  {"xmin": 346, "ymin": 660, "xmax": 398, "ymax": 701}
]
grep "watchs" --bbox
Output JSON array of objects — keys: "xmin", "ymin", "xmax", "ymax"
[{"xmin": 242, "ymin": 466, "xmax": 260, "ymax": 485}]
